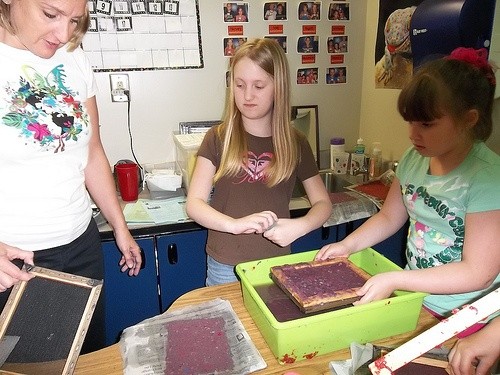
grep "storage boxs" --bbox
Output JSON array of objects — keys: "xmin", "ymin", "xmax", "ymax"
[
  {"xmin": 144, "ymin": 160, "xmax": 183, "ymax": 191},
  {"xmin": 235, "ymin": 247, "xmax": 430, "ymax": 365},
  {"xmin": 173, "ymin": 134, "xmax": 203, "ymax": 195}
]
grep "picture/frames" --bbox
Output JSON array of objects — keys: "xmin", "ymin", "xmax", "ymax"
[
  {"xmin": 180, "ymin": 121, "xmax": 221, "ymax": 133},
  {"xmin": 291, "ymin": 105, "xmax": 320, "ymax": 169}
]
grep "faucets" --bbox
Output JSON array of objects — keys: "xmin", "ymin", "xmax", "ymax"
[{"xmin": 353, "ymin": 164, "xmax": 369, "ymax": 176}]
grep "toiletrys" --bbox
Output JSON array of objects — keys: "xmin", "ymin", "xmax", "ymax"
[
  {"xmin": 355, "ymin": 138, "xmax": 366, "ymax": 154},
  {"xmin": 369, "ymin": 142, "xmax": 383, "ymax": 180},
  {"xmin": 330, "ymin": 138, "xmax": 345, "ymax": 170}
]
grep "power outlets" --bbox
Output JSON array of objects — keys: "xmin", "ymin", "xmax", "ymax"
[{"xmin": 110, "ymin": 74, "xmax": 130, "ymax": 102}]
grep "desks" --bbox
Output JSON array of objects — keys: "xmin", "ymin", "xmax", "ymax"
[{"xmin": 0, "ymin": 280, "xmax": 494, "ymax": 375}]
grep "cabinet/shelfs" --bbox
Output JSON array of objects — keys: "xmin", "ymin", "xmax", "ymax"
[
  {"xmin": 101, "ymin": 229, "xmax": 208, "ymax": 349},
  {"xmin": 292, "ymin": 217, "xmax": 402, "ymax": 267}
]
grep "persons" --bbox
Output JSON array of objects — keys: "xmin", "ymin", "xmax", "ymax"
[
  {"xmin": 330, "ymin": 4, "xmax": 344, "ymax": 20},
  {"xmin": 224, "ymin": 38, "xmax": 244, "ymax": 55},
  {"xmin": 224, "ymin": 3, "xmax": 246, "ymax": 22},
  {"xmin": 329, "ymin": 36, "xmax": 347, "ymax": 52},
  {"xmin": 185, "ymin": 39, "xmax": 333, "ymax": 287},
  {"xmin": 326, "ymin": 68, "xmax": 345, "ymax": 83},
  {"xmin": 300, "ymin": 4, "xmax": 320, "ymax": 20},
  {"xmin": 314, "ymin": 47, "xmax": 500, "ymax": 306},
  {"xmin": 447, "ymin": 315, "xmax": 500, "ymax": 375},
  {"xmin": 298, "ymin": 69, "xmax": 318, "ymax": 84},
  {"xmin": 302, "ymin": 37, "xmax": 313, "ymax": 48},
  {"xmin": 0, "ymin": 0, "xmax": 142, "ymax": 363},
  {"xmin": 265, "ymin": 4, "xmax": 285, "ymax": 20}
]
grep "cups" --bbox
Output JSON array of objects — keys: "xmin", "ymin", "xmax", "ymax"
[{"xmin": 116, "ymin": 163, "xmax": 138, "ymax": 202}]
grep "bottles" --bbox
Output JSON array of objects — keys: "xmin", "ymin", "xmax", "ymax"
[
  {"xmin": 349, "ymin": 138, "xmax": 370, "ymax": 175},
  {"xmin": 330, "ymin": 137, "xmax": 348, "ymax": 175}
]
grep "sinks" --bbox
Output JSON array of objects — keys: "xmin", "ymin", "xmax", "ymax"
[{"xmin": 320, "ymin": 173, "xmax": 354, "ymax": 193}]
